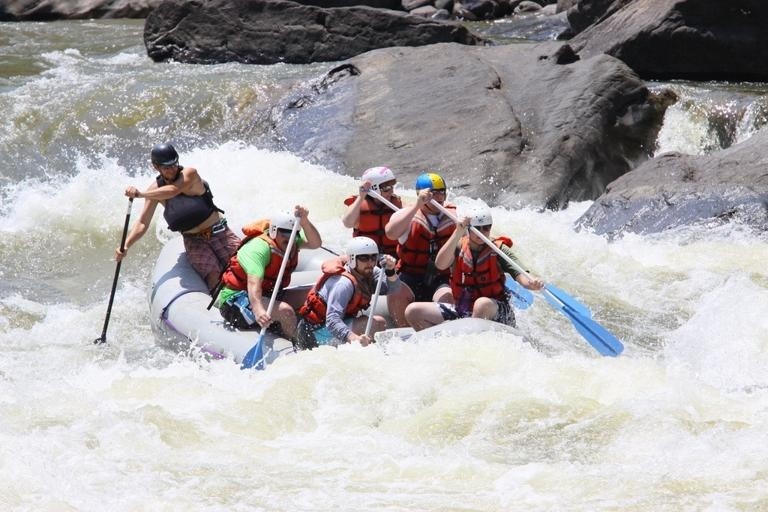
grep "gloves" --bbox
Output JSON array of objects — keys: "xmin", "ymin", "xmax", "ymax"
[{"xmin": 141, "ymin": 212, "xmax": 534, "ymax": 372}]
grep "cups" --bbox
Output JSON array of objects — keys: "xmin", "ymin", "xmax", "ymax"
[
  {"xmin": 380, "ymin": 184, "xmax": 394, "ymax": 191},
  {"xmin": 473, "ymin": 225, "xmax": 492, "ymax": 231},
  {"xmin": 431, "ymin": 189, "xmax": 444, "ymax": 194}
]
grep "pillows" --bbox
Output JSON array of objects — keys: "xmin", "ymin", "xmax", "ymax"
[
  {"xmin": 415, "ymin": 172, "xmax": 446, "ymax": 197},
  {"xmin": 345, "ymin": 236, "xmax": 379, "ymax": 270},
  {"xmin": 268, "ymin": 211, "xmax": 300, "ymax": 240},
  {"xmin": 151, "ymin": 143, "xmax": 178, "ymax": 166},
  {"xmin": 457, "ymin": 202, "xmax": 493, "ymax": 226},
  {"xmin": 361, "ymin": 166, "xmax": 396, "ymax": 196}
]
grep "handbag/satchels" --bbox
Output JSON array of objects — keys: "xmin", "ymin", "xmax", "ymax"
[{"xmin": 383, "ymin": 268, "xmax": 396, "ymax": 279}]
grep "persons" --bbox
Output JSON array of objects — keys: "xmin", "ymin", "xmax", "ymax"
[
  {"xmin": 293, "ymin": 234, "xmax": 416, "ymax": 353},
  {"xmin": 213, "ymin": 202, "xmax": 322, "ymax": 347},
  {"xmin": 340, "ymin": 166, "xmax": 406, "ymax": 269},
  {"xmin": 111, "ymin": 141, "xmax": 241, "ymax": 299},
  {"xmin": 383, "ymin": 172, "xmax": 458, "ymax": 302},
  {"xmin": 403, "ymin": 202, "xmax": 545, "ymax": 333}
]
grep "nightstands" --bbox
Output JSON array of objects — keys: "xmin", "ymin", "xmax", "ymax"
[
  {"xmin": 360, "ymin": 186, "xmax": 533, "ymax": 309},
  {"xmin": 429, "ymin": 200, "xmax": 624, "ymax": 357},
  {"xmin": 240, "ymin": 216, "xmax": 301, "ymax": 370}
]
